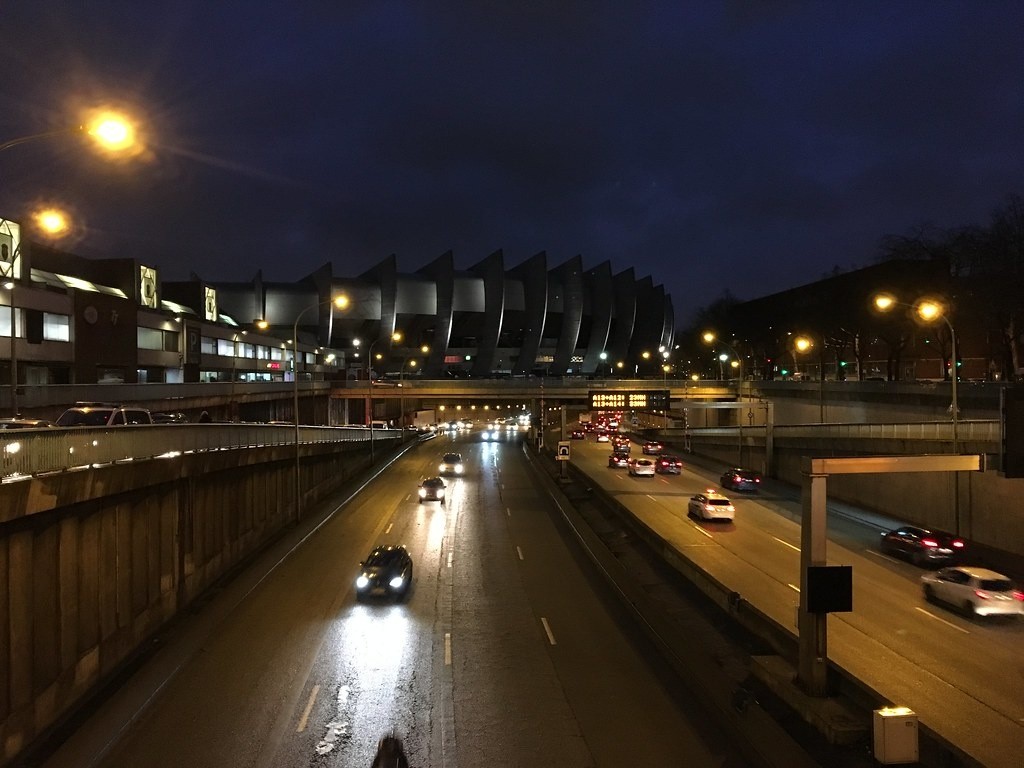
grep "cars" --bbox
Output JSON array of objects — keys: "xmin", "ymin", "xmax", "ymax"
[
  {"xmin": 687, "ymin": 493, "xmax": 737, "ymax": 524},
  {"xmin": 720, "ymin": 468, "xmax": 761, "ymax": 491},
  {"xmin": 440, "ymin": 453, "xmax": 463, "ymax": 475},
  {"xmin": 642, "ymin": 441, "xmax": 663, "ymax": 454},
  {"xmin": 416, "ymin": 478, "xmax": 449, "ymax": 503},
  {"xmin": 881, "ymin": 524, "xmax": 965, "ymax": 567},
  {"xmin": 365, "ymin": 412, "xmax": 531, "ymax": 441},
  {"xmin": 614, "ymin": 439, "xmax": 631, "ymax": 452},
  {"xmin": 569, "ymin": 410, "xmax": 637, "ymax": 444},
  {"xmin": 1, "ymin": 399, "xmax": 364, "ymax": 473},
  {"xmin": 354, "ymin": 545, "xmax": 412, "ymax": 600},
  {"xmin": 655, "ymin": 455, "xmax": 682, "ymax": 475},
  {"xmin": 609, "ymin": 452, "xmax": 631, "ymax": 468},
  {"xmin": 629, "ymin": 458, "xmax": 655, "ymax": 476},
  {"xmin": 919, "ymin": 566, "xmax": 1023, "ymax": 623}
]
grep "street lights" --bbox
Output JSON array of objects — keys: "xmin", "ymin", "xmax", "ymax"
[
  {"xmin": 795, "ymin": 333, "xmax": 824, "ymax": 423},
  {"xmin": 400, "ymin": 346, "xmax": 428, "ymax": 456},
  {"xmin": 600, "ymin": 345, "xmax": 700, "ymax": 451},
  {"xmin": 293, "ymin": 295, "xmax": 351, "ymax": 524},
  {"xmin": 231, "ymin": 320, "xmax": 269, "ymax": 396},
  {"xmin": 367, "ymin": 332, "xmax": 405, "ymax": 465},
  {"xmin": 702, "ymin": 333, "xmax": 743, "ymax": 469},
  {"xmin": 872, "ymin": 295, "xmax": 962, "ymax": 536}
]
